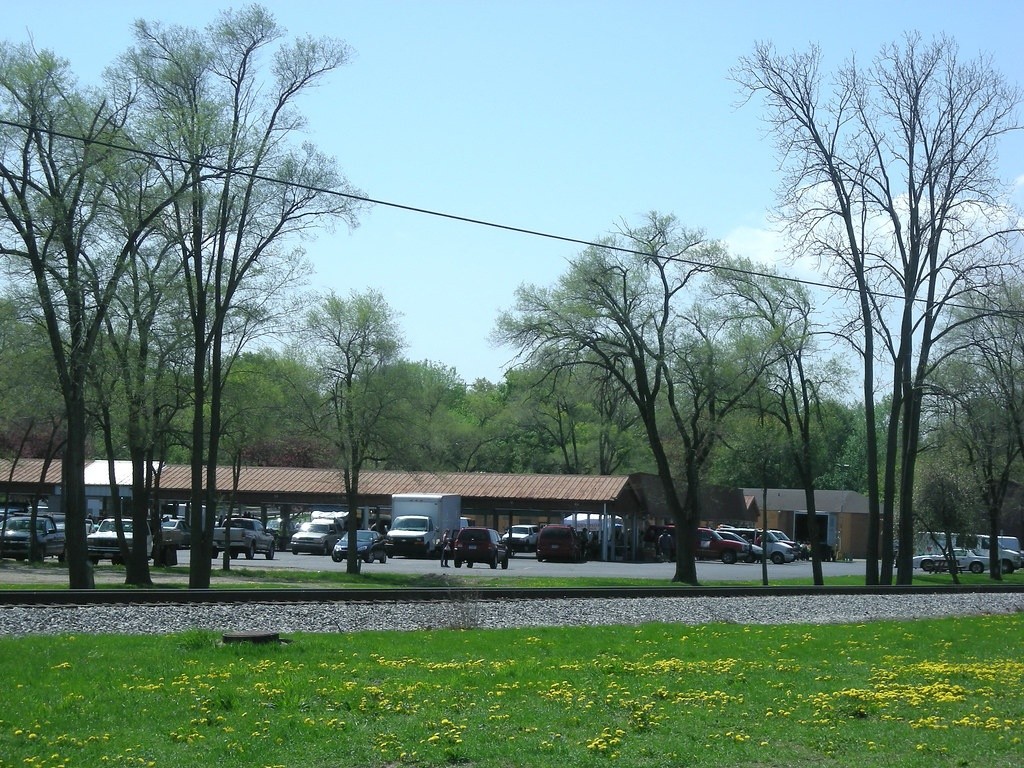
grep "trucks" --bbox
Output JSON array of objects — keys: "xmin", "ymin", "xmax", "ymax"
[
  {"xmin": 385, "ymin": 493, "xmax": 461, "ymax": 559},
  {"xmin": 793, "ymin": 510, "xmax": 837, "ymax": 561}
]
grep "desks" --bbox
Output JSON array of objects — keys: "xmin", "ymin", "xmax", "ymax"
[{"xmin": 930, "ymin": 559, "xmax": 962, "ymax": 574}]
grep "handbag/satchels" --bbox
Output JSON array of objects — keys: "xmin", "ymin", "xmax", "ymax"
[{"xmin": 444, "ymin": 544, "xmax": 451, "ymax": 551}]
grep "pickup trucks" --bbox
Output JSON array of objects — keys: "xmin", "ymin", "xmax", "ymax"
[
  {"xmin": 212, "ymin": 518, "xmax": 275, "ymax": 560},
  {"xmin": 290, "ymin": 506, "xmax": 372, "ymax": 556}
]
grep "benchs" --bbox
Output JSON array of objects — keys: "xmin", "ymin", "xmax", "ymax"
[
  {"xmin": 926, "ymin": 564, "xmax": 939, "ymax": 568},
  {"xmin": 956, "ymin": 564, "xmax": 966, "ymax": 569}
]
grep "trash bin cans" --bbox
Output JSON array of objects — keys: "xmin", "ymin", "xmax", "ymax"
[{"xmin": 162, "ymin": 540, "xmax": 177, "ymax": 566}]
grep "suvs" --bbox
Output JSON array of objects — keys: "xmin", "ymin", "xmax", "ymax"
[
  {"xmin": 450, "ymin": 527, "xmax": 508, "ymax": 569},
  {"xmin": 537, "ymin": 524, "xmax": 582, "ymax": 563}
]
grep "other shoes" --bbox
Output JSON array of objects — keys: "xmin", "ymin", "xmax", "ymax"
[{"xmin": 441, "ymin": 564, "xmax": 449, "ymax": 567}]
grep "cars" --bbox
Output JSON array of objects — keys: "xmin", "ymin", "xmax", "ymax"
[
  {"xmin": 640, "ymin": 524, "xmax": 800, "ymax": 565},
  {"xmin": 332, "ymin": 530, "xmax": 386, "ymax": 563},
  {"xmin": 0, "ymin": 507, "xmax": 191, "ymax": 565},
  {"xmin": 502, "ymin": 525, "xmax": 539, "ymax": 552},
  {"xmin": 266, "ymin": 517, "xmax": 298, "ymax": 542},
  {"xmin": 913, "ymin": 531, "xmax": 1024, "ymax": 573}
]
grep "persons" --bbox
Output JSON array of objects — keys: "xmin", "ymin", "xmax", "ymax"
[
  {"xmin": 244, "ymin": 512, "xmax": 251, "ymax": 518},
  {"xmin": 638, "ymin": 530, "xmax": 645, "ymax": 544},
  {"xmin": 742, "ymin": 534, "xmax": 748, "ymax": 542},
  {"xmin": 756, "ymin": 535, "xmax": 763, "ymax": 564},
  {"xmin": 102, "ymin": 511, "xmax": 106, "ymax": 516},
  {"xmin": 893, "ymin": 546, "xmax": 899, "ymax": 568},
  {"xmin": 441, "ymin": 528, "xmax": 451, "ymax": 567},
  {"xmin": 219, "ymin": 515, "xmax": 223, "ymax": 527},
  {"xmin": 658, "ymin": 529, "xmax": 672, "ymax": 563},
  {"xmin": 581, "ymin": 528, "xmax": 599, "ymax": 561},
  {"xmin": 23, "ymin": 500, "xmax": 30, "ymax": 513}
]
